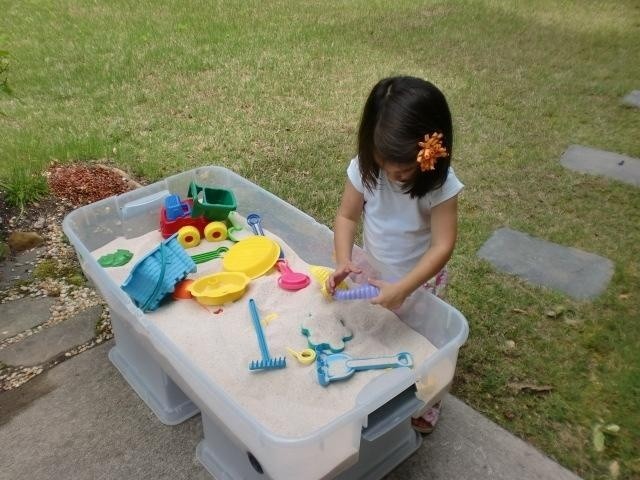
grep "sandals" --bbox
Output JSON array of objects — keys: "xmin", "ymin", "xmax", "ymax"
[{"xmin": 411, "ymin": 400, "xmax": 442, "ymax": 433}]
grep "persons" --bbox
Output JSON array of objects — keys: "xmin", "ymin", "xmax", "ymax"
[{"xmin": 324, "ymin": 74, "xmax": 465, "ymax": 434}]
